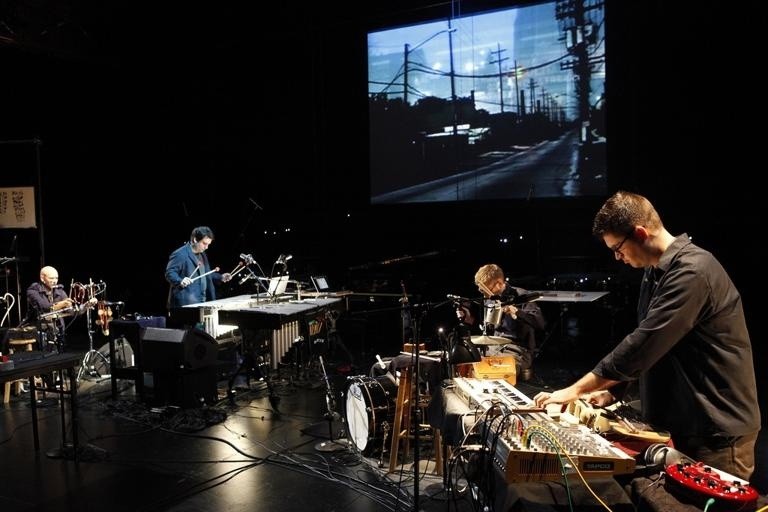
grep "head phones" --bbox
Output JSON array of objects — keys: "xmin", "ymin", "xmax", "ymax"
[{"xmin": 613, "ymin": 442, "xmax": 683, "ymax": 482}]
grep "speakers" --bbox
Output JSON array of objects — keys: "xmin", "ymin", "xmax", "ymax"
[
  {"xmin": 153, "ymin": 365, "xmax": 217, "ymax": 409},
  {"xmin": 109, "ymin": 316, "xmax": 166, "ymax": 381},
  {"xmin": 141, "ymin": 326, "xmax": 219, "ymax": 370},
  {"xmin": 93, "ymin": 337, "xmax": 134, "ymax": 377}
]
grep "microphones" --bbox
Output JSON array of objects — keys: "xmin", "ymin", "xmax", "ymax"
[
  {"xmin": 52, "ymin": 284, "xmax": 64, "ymax": 289},
  {"xmin": 513, "ymin": 292, "xmax": 539, "ymax": 305},
  {"xmin": 446, "ymin": 294, "xmax": 482, "ymax": 306},
  {"xmin": 275, "ymin": 254, "xmax": 293, "ymax": 264},
  {"xmin": 438, "ymin": 248, "xmax": 457, "ymax": 254},
  {"xmin": 239, "ymin": 253, "xmax": 256, "ymax": 264},
  {"xmin": 332, "ymin": 412, "xmax": 341, "ymax": 423},
  {"xmin": 101, "ymin": 300, "xmax": 123, "ymax": 306},
  {"xmin": 83, "ymin": 298, "xmax": 98, "ymax": 309}
]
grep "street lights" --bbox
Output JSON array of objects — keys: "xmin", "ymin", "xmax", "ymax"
[{"xmin": 404, "ymin": 28, "xmax": 460, "ymax": 105}]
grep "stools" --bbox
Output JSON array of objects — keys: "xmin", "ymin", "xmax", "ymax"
[{"xmin": 4, "ymin": 338, "xmax": 38, "ymax": 403}]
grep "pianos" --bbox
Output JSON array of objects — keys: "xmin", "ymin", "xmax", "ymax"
[{"xmin": 452, "ymin": 377, "xmax": 538, "ymax": 414}]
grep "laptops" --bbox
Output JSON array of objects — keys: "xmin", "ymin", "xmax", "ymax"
[
  {"xmin": 251, "ymin": 275, "xmax": 289, "ymax": 298},
  {"xmin": 311, "ymin": 275, "xmax": 350, "ymax": 294}
]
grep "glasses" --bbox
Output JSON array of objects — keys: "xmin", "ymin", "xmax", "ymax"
[{"xmin": 610, "ymin": 234, "xmax": 629, "ymax": 255}]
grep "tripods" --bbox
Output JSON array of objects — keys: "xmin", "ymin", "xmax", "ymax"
[{"xmin": 77, "ymin": 309, "xmax": 109, "ymax": 382}]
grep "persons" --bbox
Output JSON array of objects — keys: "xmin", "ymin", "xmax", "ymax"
[
  {"xmin": 534, "ymin": 189, "xmax": 762, "ymax": 483},
  {"xmin": 22, "ymin": 264, "xmax": 72, "ymax": 342},
  {"xmin": 454, "ymin": 263, "xmax": 547, "ymax": 361},
  {"xmin": 164, "ymin": 224, "xmax": 235, "ymax": 311}
]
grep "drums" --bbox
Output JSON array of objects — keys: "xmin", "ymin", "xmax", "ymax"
[{"xmin": 345, "ymin": 349, "xmax": 450, "ymax": 454}]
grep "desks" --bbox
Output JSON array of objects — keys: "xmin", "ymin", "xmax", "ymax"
[{"xmin": 526, "ymin": 291, "xmax": 610, "ymax": 303}]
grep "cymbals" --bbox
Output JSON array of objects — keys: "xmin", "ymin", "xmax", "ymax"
[
  {"xmin": 447, "ymin": 295, "xmax": 480, "ymax": 305},
  {"xmin": 463, "ymin": 336, "xmax": 512, "ymax": 345}
]
